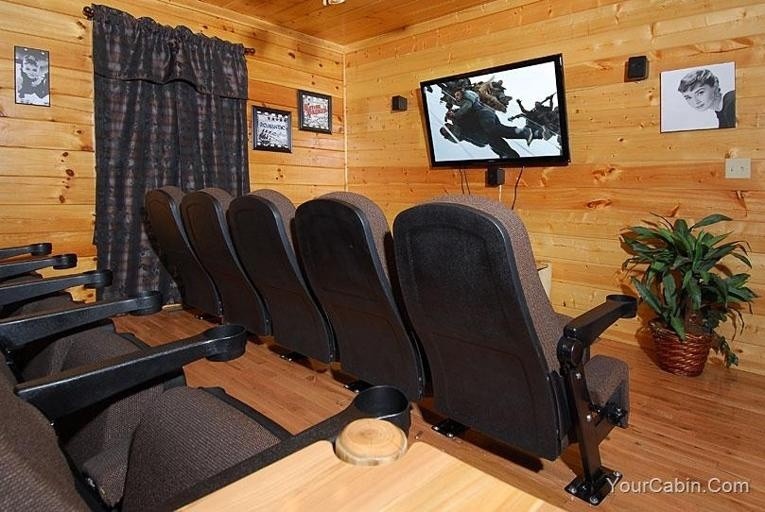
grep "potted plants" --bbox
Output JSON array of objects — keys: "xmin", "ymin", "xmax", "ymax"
[{"xmin": 621, "ymin": 211, "xmax": 762, "ymax": 377}]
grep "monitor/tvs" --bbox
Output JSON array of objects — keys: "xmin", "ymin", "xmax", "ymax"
[{"xmin": 420, "ymin": 52, "xmax": 571, "ymax": 169}]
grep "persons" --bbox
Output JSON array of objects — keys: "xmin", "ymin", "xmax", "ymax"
[
  {"xmin": 16, "ymin": 56, "xmax": 49, "ymax": 104},
  {"xmin": 678, "ymin": 69, "xmax": 736, "ymax": 128},
  {"xmin": 425, "ymin": 77, "xmax": 564, "ymax": 159}
]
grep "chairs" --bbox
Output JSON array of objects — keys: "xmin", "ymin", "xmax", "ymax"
[{"xmin": 0, "ymin": 185, "xmax": 636, "ymax": 511}]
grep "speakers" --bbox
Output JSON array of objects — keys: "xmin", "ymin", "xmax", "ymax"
[
  {"xmin": 487, "ymin": 168, "xmax": 505, "ymax": 186},
  {"xmin": 392, "ymin": 95, "xmax": 407, "ymax": 112},
  {"xmin": 627, "ymin": 56, "xmax": 649, "ymax": 81}
]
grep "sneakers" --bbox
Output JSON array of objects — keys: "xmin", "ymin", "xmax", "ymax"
[
  {"xmin": 522, "ymin": 127, "xmax": 534, "ymax": 147},
  {"xmin": 439, "ymin": 123, "xmax": 464, "ymax": 143},
  {"xmin": 538, "ymin": 126, "xmax": 550, "ymax": 140}
]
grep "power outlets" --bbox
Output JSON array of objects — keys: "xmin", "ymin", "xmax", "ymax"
[{"xmin": 725, "ymin": 159, "xmax": 751, "ymax": 178}]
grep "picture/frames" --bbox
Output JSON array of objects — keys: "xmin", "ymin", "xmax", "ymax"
[
  {"xmin": 298, "ymin": 89, "xmax": 332, "ymax": 135},
  {"xmin": 252, "ymin": 105, "xmax": 292, "ymax": 154},
  {"xmin": 14, "ymin": 45, "xmax": 51, "ymax": 107}
]
